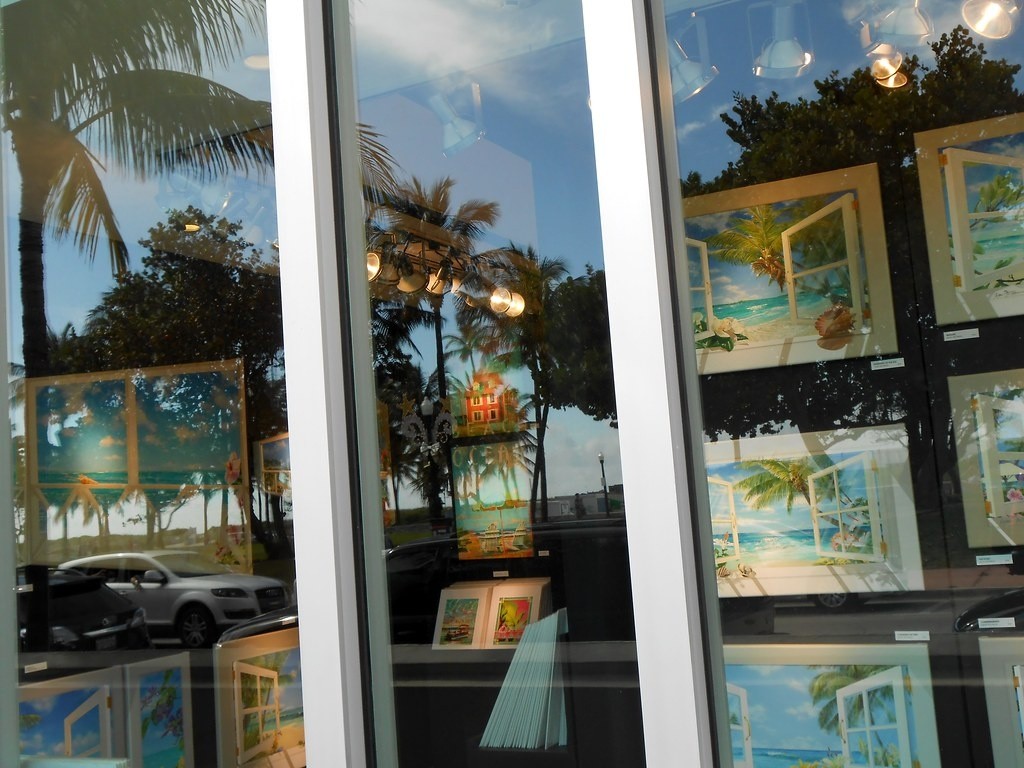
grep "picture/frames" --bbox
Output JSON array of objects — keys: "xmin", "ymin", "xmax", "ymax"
[
  {"xmin": 121, "ymin": 650, "xmax": 194, "ymax": 768},
  {"xmin": 723, "ymin": 642, "xmax": 941, "ymax": 768},
  {"xmin": 210, "ymin": 627, "xmax": 307, "ymax": 768},
  {"xmin": 16, "ymin": 662, "xmax": 125, "ymax": 758},
  {"xmin": 913, "ymin": 111, "xmax": 1024, "ymax": 326},
  {"xmin": 682, "ymin": 162, "xmax": 899, "ymax": 375},
  {"xmin": 979, "ymin": 636, "xmax": 1024, "ymax": 768},
  {"xmin": 946, "ymin": 366, "xmax": 1024, "ymax": 548},
  {"xmin": 704, "ymin": 422, "xmax": 925, "ymax": 599},
  {"xmin": 16, "ymin": 357, "xmax": 254, "ymax": 574}
]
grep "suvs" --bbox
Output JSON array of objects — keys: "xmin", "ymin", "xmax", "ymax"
[
  {"xmin": 384, "ymin": 512, "xmax": 901, "ymax": 642},
  {"xmin": 14, "ymin": 547, "xmax": 288, "ymax": 652}
]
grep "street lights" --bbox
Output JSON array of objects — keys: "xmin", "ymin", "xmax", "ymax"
[
  {"xmin": 427, "ymin": 292, "xmax": 450, "ymax": 432},
  {"xmin": 598, "ymin": 451, "xmax": 609, "ymax": 517}
]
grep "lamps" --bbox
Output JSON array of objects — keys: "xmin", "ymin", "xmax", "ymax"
[
  {"xmin": 840, "ymin": 0, "xmax": 936, "ymax": 88},
  {"xmin": 745, "ymin": 0, "xmax": 817, "ymax": 80},
  {"xmin": 667, "ymin": 13, "xmax": 719, "ymax": 107},
  {"xmin": 366, "ymin": 224, "xmax": 525, "ymax": 318},
  {"xmin": 428, "ymin": 79, "xmax": 486, "ymax": 160},
  {"xmin": 960, "ymin": 0, "xmax": 1024, "ymax": 41},
  {"xmin": 153, "ymin": 164, "xmax": 279, "ymax": 252}
]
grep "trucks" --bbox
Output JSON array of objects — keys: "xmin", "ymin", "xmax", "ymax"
[{"xmin": 529, "ymin": 501, "xmax": 574, "ymax": 518}]
